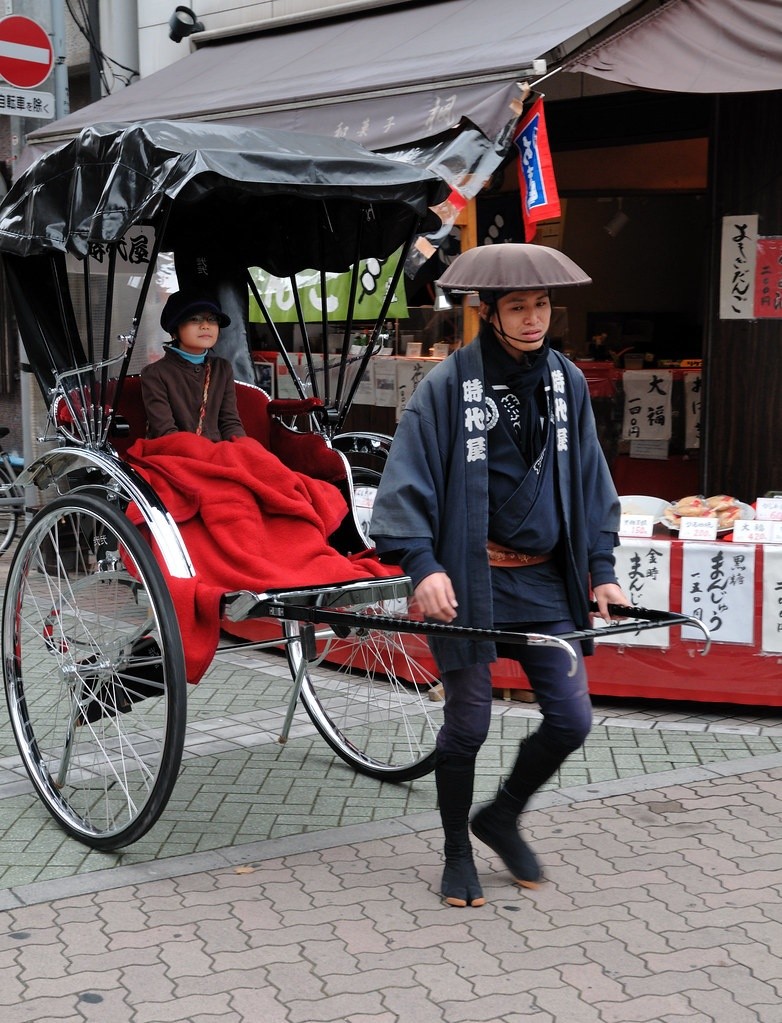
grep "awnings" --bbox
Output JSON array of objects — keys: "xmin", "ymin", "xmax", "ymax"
[{"xmin": 24, "ymin": 0, "xmax": 782, "ymax": 151}]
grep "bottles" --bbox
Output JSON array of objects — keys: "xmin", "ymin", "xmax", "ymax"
[{"xmin": 352, "ymin": 322, "xmax": 394, "ymax": 347}]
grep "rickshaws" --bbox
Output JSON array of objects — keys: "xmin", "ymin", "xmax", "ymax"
[{"xmin": 0, "ymin": 115, "xmax": 704, "ymax": 854}]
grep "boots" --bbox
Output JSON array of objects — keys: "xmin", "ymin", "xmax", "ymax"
[
  {"xmin": 471, "ymin": 733, "xmax": 569, "ymax": 888},
  {"xmin": 434, "ymin": 747, "xmax": 484, "ymax": 907}
]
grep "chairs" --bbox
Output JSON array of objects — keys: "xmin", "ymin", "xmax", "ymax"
[{"xmin": 52, "ymin": 376, "xmax": 373, "ymax": 572}]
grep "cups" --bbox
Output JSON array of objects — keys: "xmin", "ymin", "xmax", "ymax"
[
  {"xmin": 401, "ymin": 335, "xmax": 414, "ymax": 353},
  {"xmin": 432, "ymin": 343, "xmax": 449, "ymax": 358},
  {"xmin": 406, "ymin": 342, "xmax": 423, "ymax": 357},
  {"xmin": 429, "ymin": 348, "xmax": 435, "ymax": 356}
]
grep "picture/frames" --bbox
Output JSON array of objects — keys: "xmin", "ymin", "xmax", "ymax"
[{"xmin": 252, "ymin": 362, "xmax": 275, "ymax": 399}]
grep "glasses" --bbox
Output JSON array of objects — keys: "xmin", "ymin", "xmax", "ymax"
[{"xmin": 181, "ymin": 314, "xmax": 219, "ymax": 324}]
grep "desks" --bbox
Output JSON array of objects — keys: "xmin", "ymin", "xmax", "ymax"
[{"xmin": 222, "ymin": 535, "xmax": 782, "ymax": 707}]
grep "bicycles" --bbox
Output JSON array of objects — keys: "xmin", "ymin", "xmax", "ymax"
[{"xmin": 0, "ymin": 427, "xmax": 28, "ymax": 558}]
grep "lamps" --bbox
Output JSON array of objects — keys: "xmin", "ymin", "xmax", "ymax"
[{"xmin": 603, "ymin": 197, "xmax": 631, "ymax": 238}]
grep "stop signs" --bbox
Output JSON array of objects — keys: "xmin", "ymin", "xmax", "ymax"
[{"xmin": 0, "ymin": 16, "xmax": 55, "ymax": 90}]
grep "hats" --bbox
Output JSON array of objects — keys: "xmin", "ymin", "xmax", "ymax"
[
  {"xmin": 434, "ymin": 243, "xmax": 591, "ymax": 290},
  {"xmin": 160, "ymin": 289, "xmax": 232, "ymax": 334}
]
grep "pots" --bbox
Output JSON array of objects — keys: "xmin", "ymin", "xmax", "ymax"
[{"xmin": 623, "ymin": 353, "xmax": 644, "ymax": 369}]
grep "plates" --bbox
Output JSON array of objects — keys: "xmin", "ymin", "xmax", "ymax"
[{"xmin": 576, "ymin": 357, "xmax": 594, "ymax": 362}]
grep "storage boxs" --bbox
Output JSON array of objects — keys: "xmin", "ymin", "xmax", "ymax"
[{"xmin": 253, "ymin": 352, "xmax": 397, "ymax": 407}]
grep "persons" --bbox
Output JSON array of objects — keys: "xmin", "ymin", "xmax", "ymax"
[
  {"xmin": 367, "ymin": 242, "xmax": 634, "ymax": 905},
  {"xmin": 140, "ymin": 290, "xmax": 247, "ymax": 444}
]
